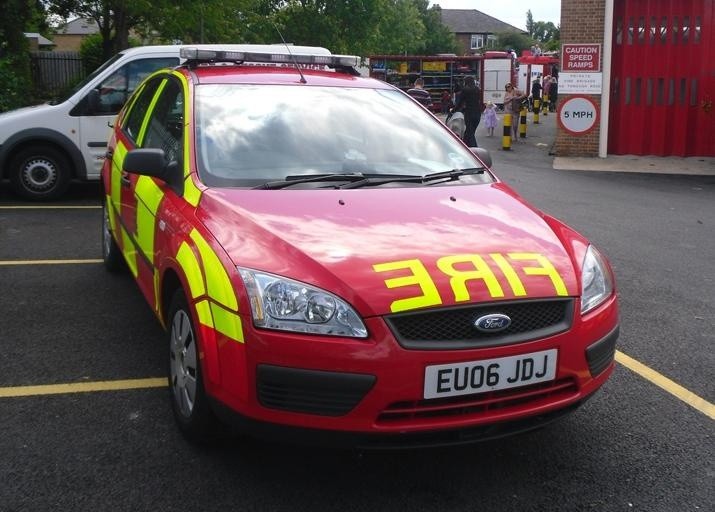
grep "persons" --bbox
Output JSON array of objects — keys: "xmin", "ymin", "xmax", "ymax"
[
  {"xmin": 406, "ymin": 76, "xmax": 433, "ymax": 111},
  {"xmin": 482, "ymin": 100, "xmax": 500, "ymax": 136},
  {"xmin": 503, "ymin": 82, "xmax": 527, "ymax": 143},
  {"xmin": 443, "ymin": 100, "xmax": 456, "ymax": 126},
  {"xmin": 440, "ymin": 44, "xmax": 558, "ymax": 113},
  {"xmin": 450, "ymin": 76, "xmax": 483, "ymax": 147}
]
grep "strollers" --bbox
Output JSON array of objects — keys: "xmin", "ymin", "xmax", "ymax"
[{"xmin": 443, "ymin": 108, "xmax": 467, "ymax": 142}]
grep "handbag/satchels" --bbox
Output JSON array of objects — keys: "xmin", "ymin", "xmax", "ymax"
[{"xmin": 512, "ymin": 96, "xmax": 524, "ymax": 112}]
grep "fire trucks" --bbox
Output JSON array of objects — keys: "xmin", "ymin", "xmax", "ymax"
[
  {"xmin": 366, "ymin": 45, "xmax": 560, "ymax": 115},
  {"xmin": 94, "ymin": 39, "xmax": 625, "ymax": 468}
]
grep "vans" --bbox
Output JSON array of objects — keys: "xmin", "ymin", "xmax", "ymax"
[{"xmin": 1, "ymin": 40, "xmax": 343, "ymax": 199}]
